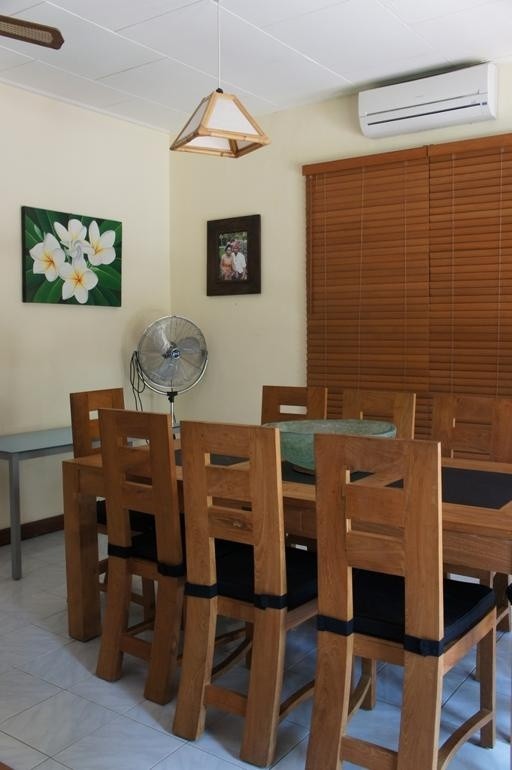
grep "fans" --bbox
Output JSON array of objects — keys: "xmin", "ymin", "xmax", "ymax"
[{"xmin": 130, "ymin": 311, "xmax": 210, "ymax": 435}]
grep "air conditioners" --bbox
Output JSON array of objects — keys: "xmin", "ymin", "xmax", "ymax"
[{"xmin": 357, "ymin": 59, "xmax": 500, "ymax": 140}]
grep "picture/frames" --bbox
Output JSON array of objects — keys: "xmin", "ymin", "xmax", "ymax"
[{"xmin": 205, "ymin": 210, "xmax": 263, "ymax": 298}]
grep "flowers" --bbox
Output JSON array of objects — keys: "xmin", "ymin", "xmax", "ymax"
[{"xmin": 29, "ymin": 214, "xmax": 118, "ymax": 307}]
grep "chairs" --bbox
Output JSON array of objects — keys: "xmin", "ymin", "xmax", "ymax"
[
  {"xmin": 67, "ymin": 386, "xmax": 158, "ymax": 623},
  {"xmin": 258, "ymin": 384, "xmax": 329, "ymax": 427},
  {"xmin": 171, "ymin": 418, "xmax": 378, "ymax": 768},
  {"xmin": 95, "ymin": 406, "xmax": 258, "ymax": 708},
  {"xmin": 342, "ymin": 388, "xmax": 417, "ymax": 438},
  {"xmin": 297, "ymin": 432, "xmax": 499, "ymax": 768},
  {"xmin": 429, "ymin": 389, "xmax": 510, "ymax": 466}
]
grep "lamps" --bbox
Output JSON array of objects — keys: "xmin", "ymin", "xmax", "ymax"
[{"xmin": 167, "ymin": 0, "xmax": 273, "ymax": 159}]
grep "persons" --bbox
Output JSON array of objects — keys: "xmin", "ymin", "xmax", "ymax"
[{"xmin": 220, "ymin": 239, "xmax": 247, "ymax": 280}]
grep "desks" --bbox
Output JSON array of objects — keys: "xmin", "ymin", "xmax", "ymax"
[
  {"xmin": 0, "ymin": 420, "xmax": 100, "ymax": 581},
  {"xmin": 63, "ymin": 429, "xmax": 510, "ymax": 645}
]
